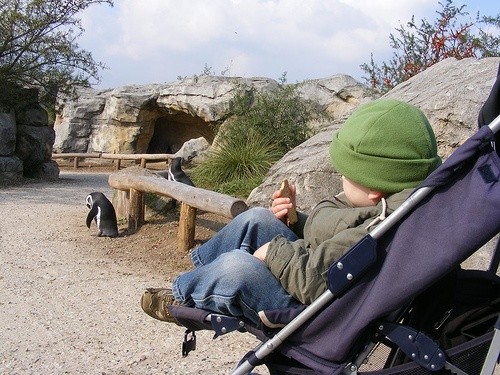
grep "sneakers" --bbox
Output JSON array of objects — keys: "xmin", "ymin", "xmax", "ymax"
[{"xmin": 140, "ymin": 287, "xmax": 184, "ymax": 326}]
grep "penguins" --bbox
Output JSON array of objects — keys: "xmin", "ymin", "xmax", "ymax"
[
  {"xmin": 169, "ymin": 157, "xmax": 196, "ymax": 187},
  {"xmin": 85, "ymin": 192, "xmax": 119, "ymax": 238}
]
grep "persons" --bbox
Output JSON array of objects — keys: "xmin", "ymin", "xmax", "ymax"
[{"xmin": 142, "ymin": 98, "xmax": 442, "ymax": 329}]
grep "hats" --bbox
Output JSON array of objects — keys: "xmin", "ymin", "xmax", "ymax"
[{"xmin": 328, "ymin": 99, "xmax": 443, "ymax": 193}]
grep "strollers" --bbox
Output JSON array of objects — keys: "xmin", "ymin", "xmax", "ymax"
[{"xmin": 162, "ymin": 62, "xmax": 500, "ymax": 375}]
